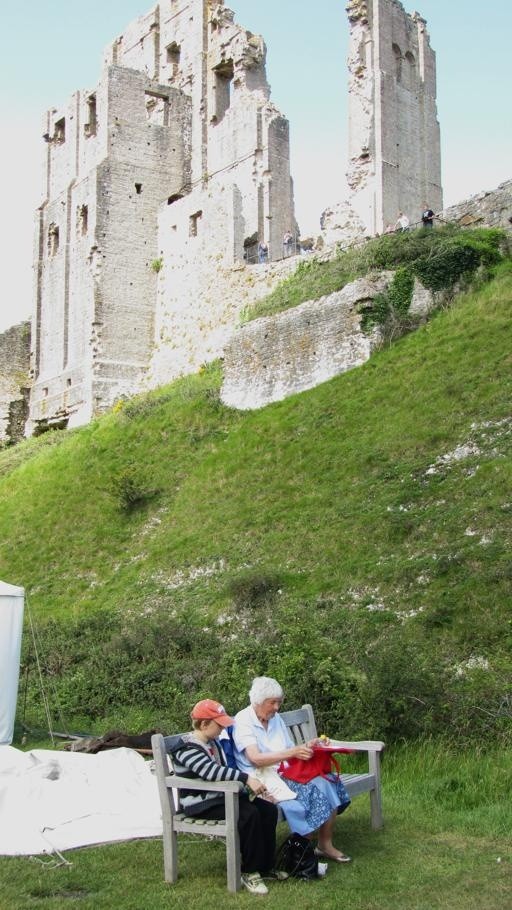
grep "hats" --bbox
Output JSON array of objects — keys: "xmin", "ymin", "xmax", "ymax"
[{"xmin": 191, "ymin": 698, "xmax": 236, "ymax": 729}]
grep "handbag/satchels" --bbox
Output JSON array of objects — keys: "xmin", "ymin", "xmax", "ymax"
[
  {"xmin": 276, "ymin": 744, "xmax": 354, "ymax": 785},
  {"xmin": 276, "ymin": 831, "xmax": 317, "ymax": 882}
]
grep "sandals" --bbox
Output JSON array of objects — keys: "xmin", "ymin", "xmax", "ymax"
[{"xmin": 314, "ymin": 845, "xmax": 351, "ymax": 862}]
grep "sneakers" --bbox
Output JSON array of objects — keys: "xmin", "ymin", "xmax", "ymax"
[{"xmin": 239, "ymin": 870, "xmax": 290, "ymax": 896}]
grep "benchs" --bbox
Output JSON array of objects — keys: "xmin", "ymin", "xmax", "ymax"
[{"xmin": 152, "ymin": 703, "xmax": 384, "ymax": 892}]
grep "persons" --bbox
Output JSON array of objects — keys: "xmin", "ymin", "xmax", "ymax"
[
  {"xmin": 284, "ymin": 231, "xmax": 292, "ymax": 258},
  {"xmin": 169, "ymin": 700, "xmax": 290, "ymax": 895},
  {"xmin": 422, "ymin": 204, "xmax": 436, "ymax": 227},
  {"xmin": 231, "ymin": 677, "xmax": 352, "ymax": 875},
  {"xmin": 395, "ymin": 212, "xmax": 409, "ymax": 233},
  {"xmin": 383, "ymin": 223, "xmax": 397, "ymax": 234},
  {"xmin": 257, "ymin": 243, "xmax": 266, "ymax": 264}
]
